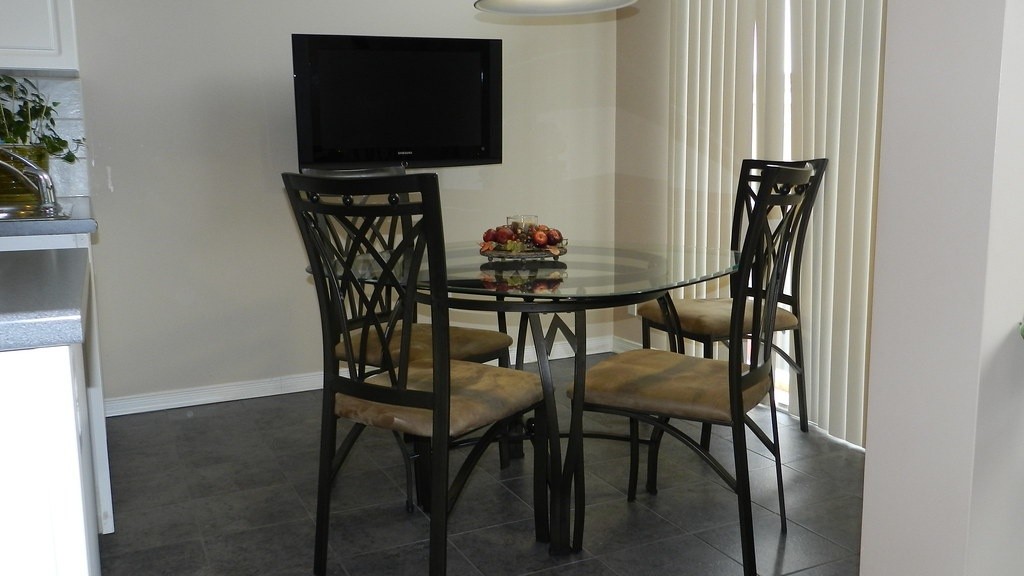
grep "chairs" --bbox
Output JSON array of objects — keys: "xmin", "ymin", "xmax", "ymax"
[
  {"xmin": 638, "ymin": 159, "xmax": 828, "ymax": 452},
  {"xmin": 301, "ymin": 166, "xmax": 513, "ymax": 507},
  {"xmin": 280, "ymin": 172, "xmax": 555, "ymax": 576},
  {"xmin": 567, "ymin": 162, "xmax": 814, "ymax": 576}
]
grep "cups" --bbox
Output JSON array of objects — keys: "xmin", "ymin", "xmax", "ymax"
[{"xmin": 507, "ymin": 215, "xmax": 538, "ymax": 243}]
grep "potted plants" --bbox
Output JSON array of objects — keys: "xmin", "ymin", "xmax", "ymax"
[{"xmin": 0, "ymin": 74, "xmax": 85, "ymax": 202}]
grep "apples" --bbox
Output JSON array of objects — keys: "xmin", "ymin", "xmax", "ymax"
[{"xmin": 483, "ymin": 224, "xmax": 563, "ymax": 248}]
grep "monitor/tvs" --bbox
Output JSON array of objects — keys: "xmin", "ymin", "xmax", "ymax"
[{"xmin": 291, "ymin": 34, "xmax": 502, "ymax": 173}]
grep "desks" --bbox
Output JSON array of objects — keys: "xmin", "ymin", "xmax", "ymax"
[{"xmin": 305, "ymin": 239, "xmax": 744, "ymax": 556}]
